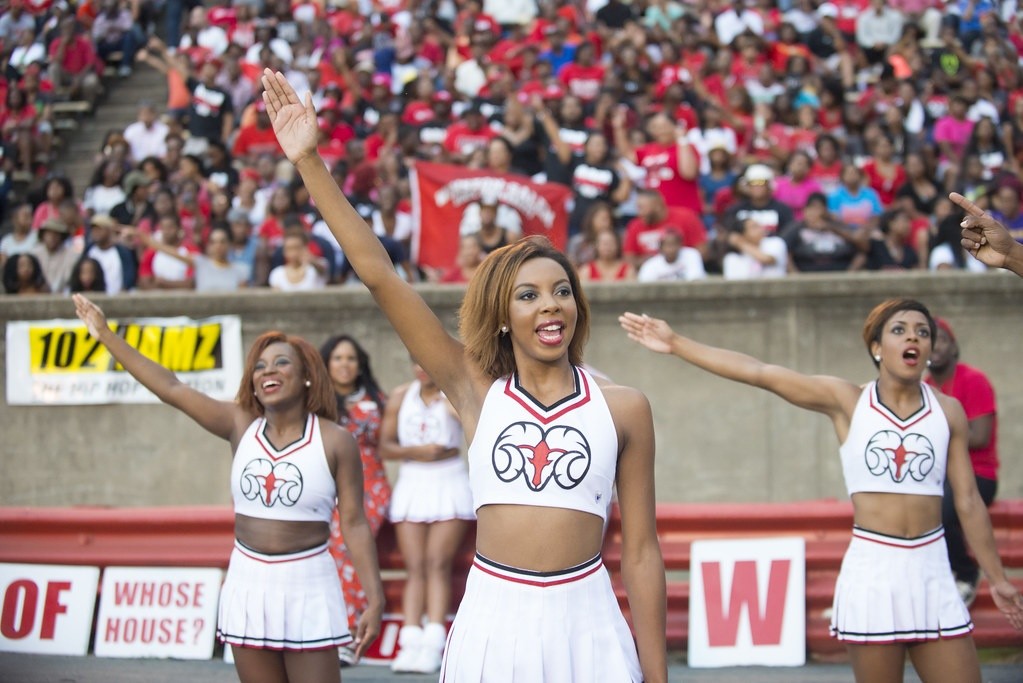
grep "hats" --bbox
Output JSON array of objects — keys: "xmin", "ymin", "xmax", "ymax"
[
  {"xmin": 90, "ymin": 215, "xmax": 115, "ymax": 234},
  {"xmin": 226, "ymin": 206, "xmax": 255, "ymax": 226},
  {"xmin": 41, "ymin": 215, "xmax": 66, "ymax": 233},
  {"xmin": 933, "ymin": 318, "xmax": 960, "ymax": 357},
  {"xmin": 737, "ymin": 164, "xmax": 777, "ymax": 197},
  {"xmin": 124, "ymin": 171, "xmax": 153, "ymax": 195}
]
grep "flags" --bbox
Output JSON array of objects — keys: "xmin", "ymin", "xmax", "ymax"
[{"xmin": 404, "ymin": 158, "xmax": 575, "ymax": 269}]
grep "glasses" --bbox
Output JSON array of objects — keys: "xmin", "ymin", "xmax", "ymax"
[{"xmin": 748, "ymin": 180, "xmax": 766, "ymax": 187}]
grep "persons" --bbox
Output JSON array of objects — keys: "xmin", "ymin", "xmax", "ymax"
[
  {"xmin": 72, "ymin": 292, "xmax": 386, "ymax": 683},
  {"xmin": 320, "ymin": 334, "xmax": 392, "ymax": 669},
  {"xmin": 948, "ymin": 191, "xmax": 1023, "ymax": 277},
  {"xmin": 618, "ymin": 298, "xmax": 1023, "ymax": 683},
  {"xmin": 260, "ymin": 63, "xmax": 668, "ymax": 683},
  {"xmin": 0, "ymin": 0, "xmax": 1023, "ymax": 296},
  {"xmin": 376, "ymin": 352, "xmax": 477, "ymax": 674}
]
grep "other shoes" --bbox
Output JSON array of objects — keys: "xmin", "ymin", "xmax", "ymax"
[
  {"xmin": 391, "ymin": 621, "xmax": 447, "ymax": 675},
  {"xmin": 337, "ymin": 646, "xmax": 355, "ymax": 666},
  {"xmin": 955, "ymin": 568, "xmax": 980, "ymax": 609}
]
